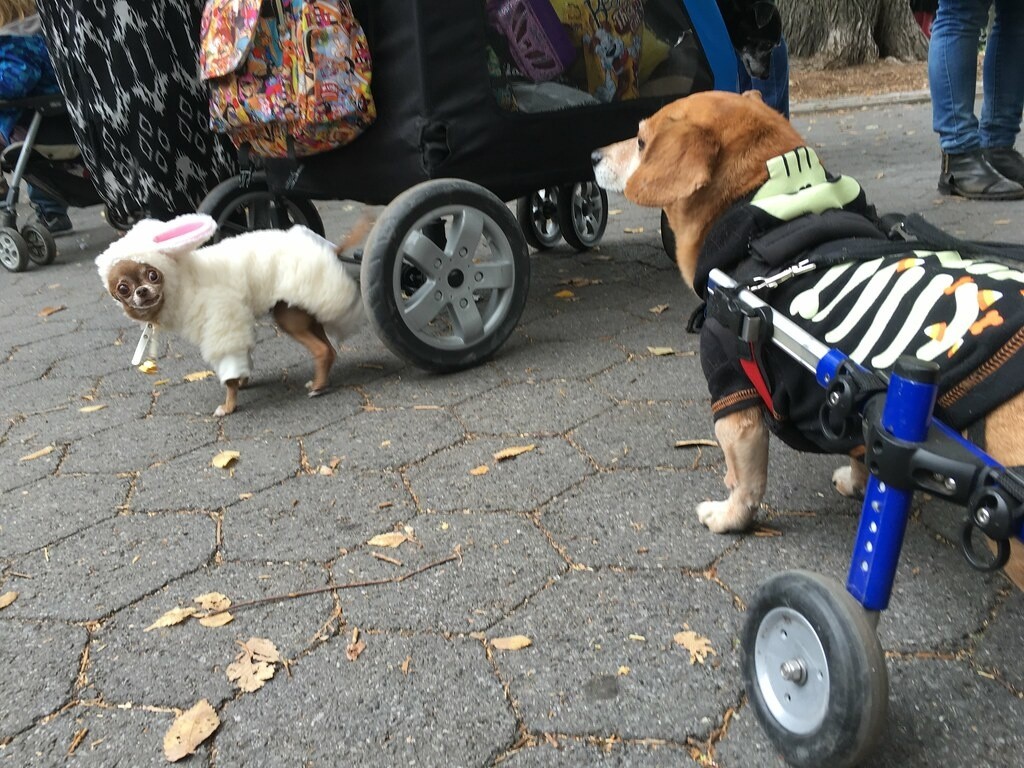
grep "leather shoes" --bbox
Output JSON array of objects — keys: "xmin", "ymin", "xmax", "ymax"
[
  {"xmin": 938, "ymin": 151, "xmax": 1024, "ymax": 201},
  {"xmin": 980, "ymin": 147, "xmax": 1024, "ymax": 187}
]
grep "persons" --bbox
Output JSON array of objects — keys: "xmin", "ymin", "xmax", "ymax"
[{"xmin": 928, "ymin": 0, "xmax": 1024, "ymax": 200}]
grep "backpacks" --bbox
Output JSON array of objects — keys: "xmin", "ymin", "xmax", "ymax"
[{"xmin": 198, "ymin": 0, "xmax": 376, "ymax": 162}]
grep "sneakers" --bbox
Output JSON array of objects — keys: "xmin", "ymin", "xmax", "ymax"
[{"xmin": 29, "ymin": 199, "xmax": 73, "ymax": 238}]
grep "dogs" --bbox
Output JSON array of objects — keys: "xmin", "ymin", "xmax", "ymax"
[
  {"xmin": 105, "ymin": 213, "xmax": 376, "ymax": 415},
  {"xmin": 592, "ymin": 89, "xmax": 1024, "ymax": 592}
]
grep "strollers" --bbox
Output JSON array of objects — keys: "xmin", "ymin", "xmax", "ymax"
[
  {"xmin": 196, "ymin": 0, "xmax": 791, "ymax": 377},
  {"xmin": 1, "ymin": 91, "xmax": 136, "ymax": 276}
]
